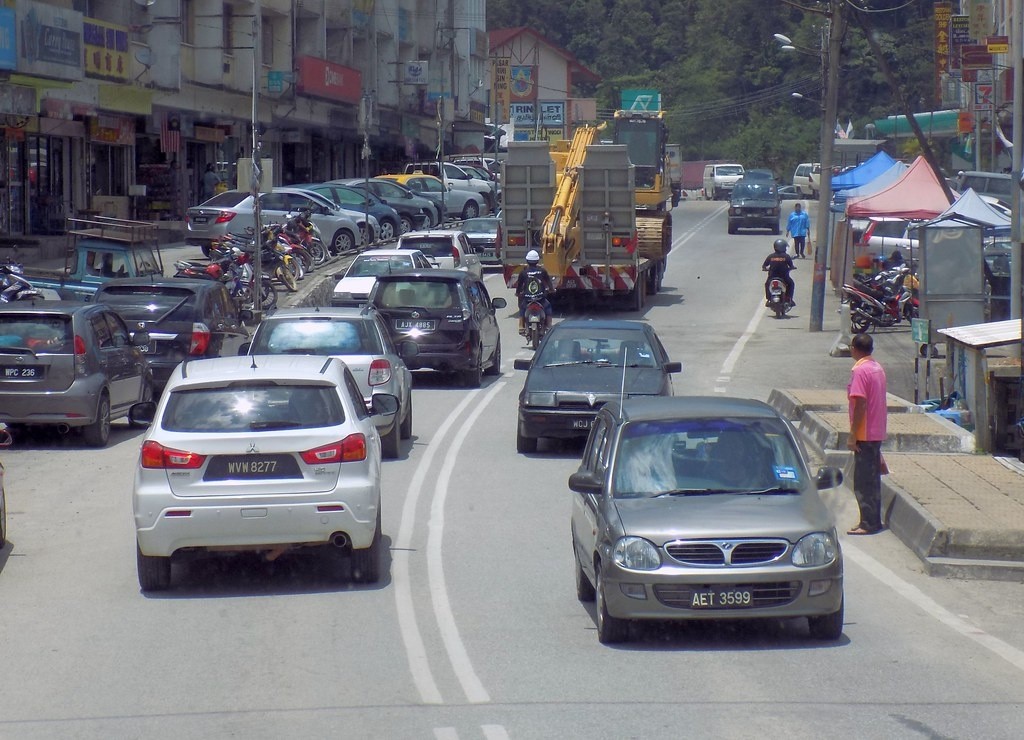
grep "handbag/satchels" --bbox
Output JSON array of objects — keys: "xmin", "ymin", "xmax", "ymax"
[{"xmin": 806, "ymin": 234, "xmax": 813, "ymax": 255}]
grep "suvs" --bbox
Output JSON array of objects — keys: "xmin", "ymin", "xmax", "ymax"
[{"xmin": 726, "ymin": 168, "xmax": 782, "ymax": 236}]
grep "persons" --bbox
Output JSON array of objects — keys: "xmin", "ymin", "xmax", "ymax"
[
  {"xmin": 706, "ymin": 427, "xmax": 768, "ymax": 493},
  {"xmin": 515, "ymin": 250, "xmax": 555, "ymax": 335},
  {"xmin": 785, "ymin": 203, "xmax": 810, "ymax": 258},
  {"xmin": 557, "ymin": 336, "xmax": 579, "ymax": 361},
  {"xmin": 884, "ymin": 251, "xmax": 906, "ymax": 271},
  {"xmin": 762, "ymin": 239, "xmax": 796, "ymax": 306},
  {"xmin": 846, "ymin": 333, "xmax": 887, "ymax": 535},
  {"xmin": 200, "ymin": 162, "xmax": 222, "ymax": 203}
]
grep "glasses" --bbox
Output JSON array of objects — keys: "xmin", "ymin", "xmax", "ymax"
[{"xmin": 848, "ymin": 345, "xmax": 854, "ymax": 351}]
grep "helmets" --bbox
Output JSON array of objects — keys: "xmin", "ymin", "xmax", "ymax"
[
  {"xmin": 230, "ymin": 247, "xmax": 241, "ymax": 259},
  {"xmin": 525, "ymin": 250, "xmax": 539, "ymax": 261},
  {"xmin": 206, "ymin": 265, "xmax": 221, "ymax": 279},
  {"xmin": 774, "ymin": 239, "xmax": 788, "ymax": 252}
]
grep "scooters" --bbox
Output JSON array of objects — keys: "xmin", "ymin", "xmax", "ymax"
[
  {"xmin": 0, "ymin": 245, "xmax": 45, "ymax": 302},
  {"xmin": 173, "ymin": 198, "xmax": 333, "ymax": 309},
  {"xmin": 841, "ymin": 243, "xmax": 921, "ymax": 335},
  {"xmin": 761, "ymin": 267, "xmax": 798, "ymax": 319}
]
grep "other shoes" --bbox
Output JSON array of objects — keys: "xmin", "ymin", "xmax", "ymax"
[
  {"xmin": 792, "ymin": 254, "xmax": 799, "ymax": 259},
  {"xmin": 800, "ymin": 252, "xmax": 805, "ymax": 258},
  {"xmin": 519, "ymin": 327, "xmax": 526, "ymax": 334},
  {"xmin": 788, "ymin": 301, "xmax": 795, "ymax": 306},
  {"xmin": 846, "ymin": 523, "xmax": 883, "ymax": 534},
  {"xmin": 766, "ymin": 300, "xmax": 771, "ymax": 307}
]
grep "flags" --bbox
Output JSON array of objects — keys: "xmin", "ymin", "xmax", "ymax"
[
  {"xmin": 835, "ymin": 118, "xmax": 855, "ymax": 139},
  {"xmin": 159, "ymin": 112, "xmax": 181, "ymax": 152}
]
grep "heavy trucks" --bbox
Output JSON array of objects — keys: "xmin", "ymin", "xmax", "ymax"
[{"xmin": 499, "ymin": 140, "xmax": 669, "ymax": 313}]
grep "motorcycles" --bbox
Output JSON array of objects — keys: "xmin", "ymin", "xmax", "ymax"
[{"xmin": 519, "ymin": 296, "xmax": 550, "ymax": 351}]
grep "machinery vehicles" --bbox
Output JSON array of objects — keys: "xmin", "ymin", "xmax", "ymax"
[{"xmin": 505, "ymin": 108, "xmax": 675, "ymax": 292}]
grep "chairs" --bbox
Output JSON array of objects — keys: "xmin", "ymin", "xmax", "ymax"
[{"xmin": 556, "ymin": 342, "xmax": 580, "ymax": 361}]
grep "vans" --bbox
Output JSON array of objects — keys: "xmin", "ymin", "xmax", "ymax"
[
  {"xmin": 793, "ymin": 163, "xmax": 821, "ymax": 199},
  {"xmin": 857, "ymin": 215, "xmax": 930, "ymax": 267},
  {"xmin": 703, "ymin": 163, "xmax": 746, "ymax": 201},
  {"xmin": 956, "ymin": 170, "xmax": 1013, "ymax": 204}
]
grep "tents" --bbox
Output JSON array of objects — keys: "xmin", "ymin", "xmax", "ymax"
[{"xmin": 826, "ymin": 150, "xmax": 1012, "ymax": 303}]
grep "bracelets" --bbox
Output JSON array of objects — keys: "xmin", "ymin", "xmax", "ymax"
[{"xmin": 563, "ymin": 353, "xmax": 564, "ymax": 358}]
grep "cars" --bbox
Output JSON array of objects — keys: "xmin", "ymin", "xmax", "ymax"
[
  {"xmin": 127, "ymin": 351, "xmax": 401, "ymax": 591},
  {"xmin": 321, "ymin": 157, "xmax": 507, "ymax": 389},
  {"xmin": 236, "ymin": 306, "xmax": 415, "ymax": 459},
  {"xmin": 979, "ymin": 195, "xmax": 1012, "ymax": 218},
  {"xmin": 776, "ymin": 185, "xmax": 799, "ymax": 199},
  {"xmin": 274, "ymin": 187, "xmax": 381, "ymax": 246},
  {"xmin": 92, "ymin": 277, "xmax": 255, "ymax": 398},
  {"xmin": 513, "ymin": 317, "xmax": 684, "ymax": 454},
  {"xmin": 284, "ymin": 183, "xmax": 402, "ymax": 242},
  {"xmin": 0, "ymin": 300, "xmax": 158, "ymax": 448},
  {"xmin": 567, "ymin": 394, "xmax": 847, "ymax": 643},
  {"xmin": 182, "ymin": 188, "xmax": 362, "ymax": 255}
]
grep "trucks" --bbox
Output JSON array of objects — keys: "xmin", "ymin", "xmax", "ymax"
[{"xmin": 0, "ymin": 215, "xmax": 164, "ymax": 303}]
